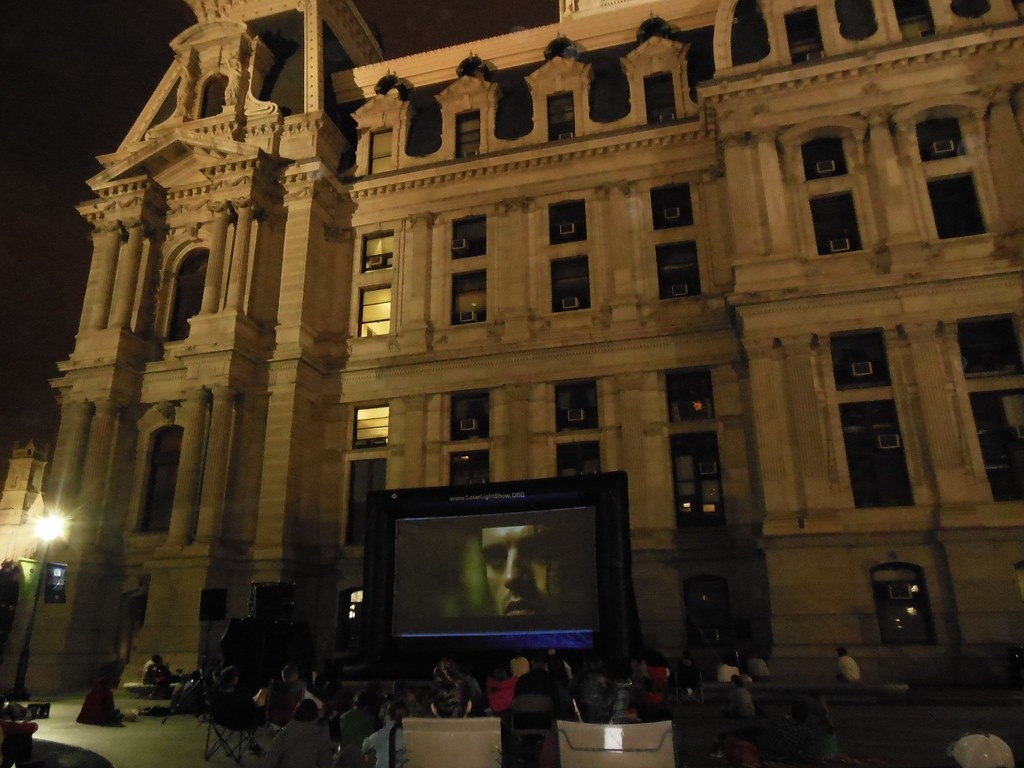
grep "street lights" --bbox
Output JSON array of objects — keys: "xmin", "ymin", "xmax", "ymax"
[{"xmin": 5, "ymin": 518, "xmax": 60, "ymax": 700}]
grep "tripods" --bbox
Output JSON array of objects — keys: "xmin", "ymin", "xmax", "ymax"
[{"xmin": 162, "ymin": 622, "xmax": 213, "ymax": 724}]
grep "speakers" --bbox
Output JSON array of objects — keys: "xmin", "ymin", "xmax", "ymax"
[{"xmin": 198, "ymin": 589, "xmax": 227, "ymax": 622}]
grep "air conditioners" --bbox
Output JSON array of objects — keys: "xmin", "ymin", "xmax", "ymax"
[
  {"xmin": 886, "ymin": 584, "xmax": 913, "ymax": 600},
  {"xmin": 560, "ymin": 223, "xmax": 578, "ymax": 235},
  {"xmin": 663, "ymin": 208, "xmax": 680, "ymax": 219},
  {"xmin": 699, "ymin": 460, "xmax": 717, "ymax": 475},
  {"xmin": 659, "ymin": 113, "xmax": 674, "ymax": 122},
  {"xmin": 671, "ymin": 283, "xmax": 688, "ymax": 296},
  {"xmin": 828, "ymin": 238, "xmax": 850, "ymax": 254},
  {"xmin": 851, "ymin": 361, "xmax": 873, "ymax": 378},
  {"xmin": 563, "ymin": 297, "xmax": 581, "ymax": 309},
  {"xmin": 930, "ymin": 139, "xmax": 955, "ymax": 159},
  {"xmin": 567, "ymin": 408, "xmax": 587, "ymax": 421},
  {"xmin": 460, "ymin": 419, "xmax": 481, "ymax": 431},
  {"xmin": 814, "ymin": 160, "xmax": 836, "ymax": 178},
  {"xmin": 369, "ymin": 256, "xmax": 387, "ymax": 267},
  {"xmin": 559, "ymin": 132, "xmax": 573, "ymax": 140},
  {"xmin": 460, "ymin": 311, "xmax": 477, "ymax": 323},
  {"xmin": 451, "ymin": 238, "xmax": 469, "ymax": 253},
  {"xmin": 877, "ymin": 433, "xmax": 900, "ymax": 450},
  {"xmin": 919, "ymin": 28, "xmax": 934, "ymax": 38},
  {"xmin": 807, "ymin": 50, "xmax": 824, "ymax": 61},
  {"xmin": 465, "ymin": 150, "xmax": 478, "ymax": 157}
]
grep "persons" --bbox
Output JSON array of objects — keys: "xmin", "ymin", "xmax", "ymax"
[
  {"xmin": 76, "ymin": 675, "xmax": 125, "ymax": 727},
  {"xmin": 142, "ymin": 653, "xmax": 179, "ymax": 700},
  {"xmin": 834, "ymin": 646, "xmax": 861, "ymax": 682},
  {"xmin": 719, "ymin": 674, "xmax": 755, "ymax": 719},
  {"xmin": 261, "ymin": 658, "xmax": 699, "ymax": 768},
  {"xmin": 478, "ymin": 524, "xmax": 562, "ymax": 617},
  {"xmin": 710, "ymin": 692, "xmax": 840, "ymax": 763}
]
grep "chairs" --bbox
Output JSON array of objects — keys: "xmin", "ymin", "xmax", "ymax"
[
  {"xmin": 557, "ymin": 719, "xmax": 676, "ymax": 768},
  {"xmin": 266, "ymin": 679, "xmax": 306, "ymax": 737},
  {"xmin": 202, "ymin": 668, "xmax": 263, "ymax": 763},
  {"xmin": 401, "ymin": 717, "xmax": 503, "ymax": 768}
]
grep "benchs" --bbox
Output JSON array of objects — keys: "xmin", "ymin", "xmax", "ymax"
[{"xmin": 123, "ymin": 683, "xmax": 178, "ymax": 700}]
